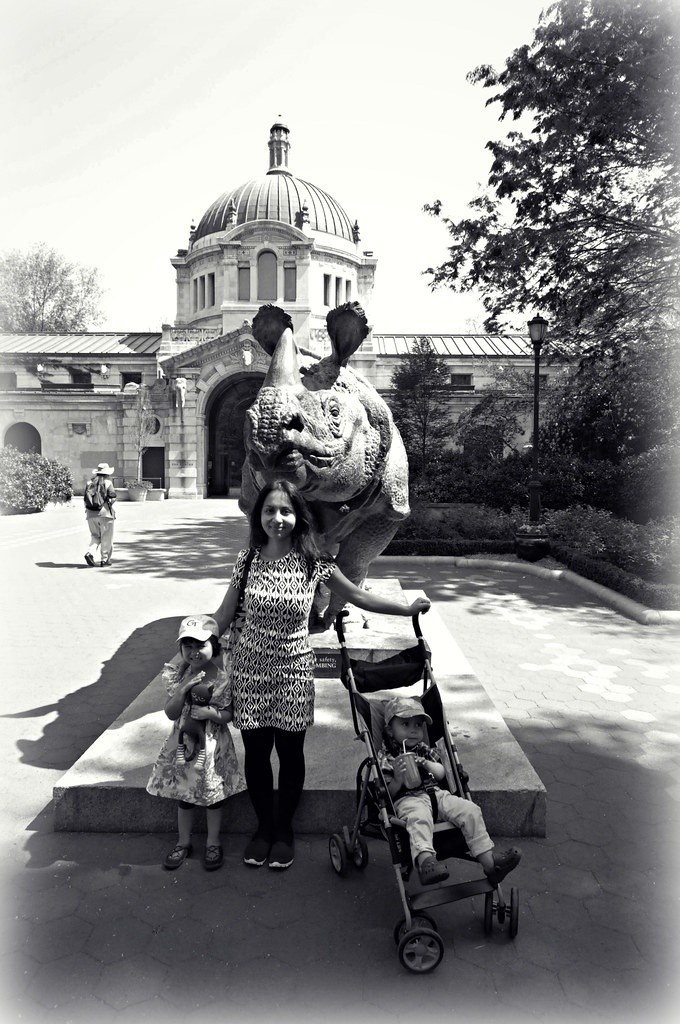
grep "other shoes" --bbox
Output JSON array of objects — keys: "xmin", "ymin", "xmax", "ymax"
[
  {"xmin": 163, "ymin": 842, "xmax": 192, "ymax": 869},
  {"xmin": 202, "ymin": 845, "xmax": 225, "ymax": 872},
  {"xmin": 84, "ymin": 552, "xmax": 96, "ymax": 567},
  {"xmin": 101, "ymin": 561, "xmax": 112, "ymax": 567}
]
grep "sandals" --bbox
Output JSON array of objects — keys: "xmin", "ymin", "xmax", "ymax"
[
  {"xmin": 418, "ymin": 856, "xmax": 449, "ymax": 886},
  {"xmin": 484, "ymin": 846, "xmax": 522, "ymax": 884}
]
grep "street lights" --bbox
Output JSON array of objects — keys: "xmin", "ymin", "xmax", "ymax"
[{"xmin": 525, "ymin": 310, "xmax": 551, "ymax": 527}]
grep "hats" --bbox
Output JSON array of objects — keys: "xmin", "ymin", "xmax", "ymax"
[
  {"xmin": 176, "ymin": 614, "xmax": 220, "ymax": 642},
  {"xmin": 384, "ymin": 696, "xmax": 433, "ymax": 725},
  {"xmin": 92, "ymin": 463, "xmax": 115, "ymax": 476}
]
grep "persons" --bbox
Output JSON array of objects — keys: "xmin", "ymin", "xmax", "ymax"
[
  {"xmin": 177, "ymin": 479, "xmax": 430, "ymax": 867},
  {"xmin": 84, "ymin": 462, "xmax": 117, "ymax": 566},
  {"xmin": 146, "ymin": 616, "xmax": 248, "ymax": 870},
  {"xmin": 378, "ymin": 697, "xmax": 522, "ymax": 886}
]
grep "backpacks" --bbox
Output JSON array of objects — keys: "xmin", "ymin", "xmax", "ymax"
[{"xmin": 84, "ymin": 476, "xmax": 112, "ymax": 515}]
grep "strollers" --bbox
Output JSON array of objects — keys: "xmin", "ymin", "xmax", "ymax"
[{"xmin": 320, "ymin": 606, "xmax": 522, "ymax": 979}]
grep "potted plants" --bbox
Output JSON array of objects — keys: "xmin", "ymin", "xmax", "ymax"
[{"xmin": 124, "ymin": 480, "xmax": 153, "ymax": 501}]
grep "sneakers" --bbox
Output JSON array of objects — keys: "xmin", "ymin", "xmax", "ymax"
[
  {"xmin": 243, "ymin": 830, "xmax": 272, "ymax": 865},
  {"xmin": 267, "ymin": 826, "xmax": 295, "ymax": 867}
]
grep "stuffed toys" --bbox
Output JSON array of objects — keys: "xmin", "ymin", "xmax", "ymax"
[{"xmin": 175, "ymin": 684, "xmax": 214, "ymax": 770}]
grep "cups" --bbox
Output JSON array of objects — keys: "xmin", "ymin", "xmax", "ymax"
[{"xmin": 392, "ymin": 752, "xmax": 421, "ymax": 789}]
